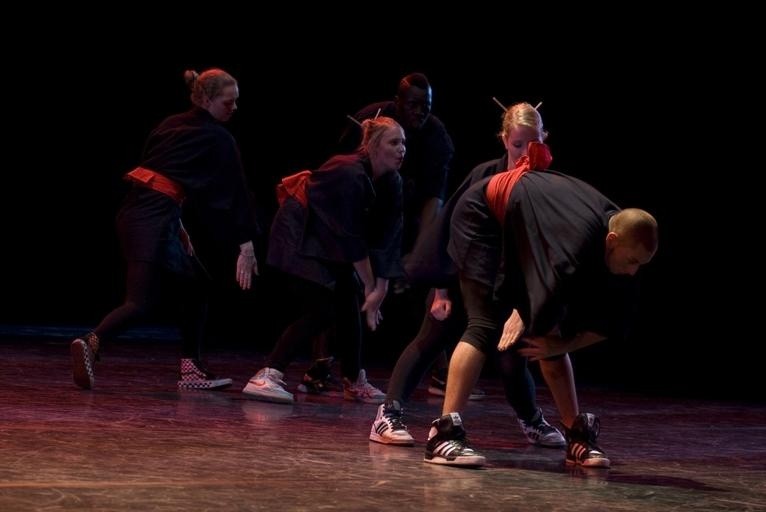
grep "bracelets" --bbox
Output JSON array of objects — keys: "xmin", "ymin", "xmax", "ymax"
[{"xmin": 240, "ymin": 251, "xmax": 254, "ymax": 259}]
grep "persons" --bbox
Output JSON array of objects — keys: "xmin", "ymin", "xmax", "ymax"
[
  {"xmin": 238, "ymin": 105, "xmax": 408, "ymax": 404},
  {"xmin": 424, "ymin": 142, "xmax": 662, "ymax": 468},
  {"xmin": 366, "ymin": 98, "xmax": 568, "ymax": 449},
  {"xmin": 294, "ymin": 68, "xmax": 485, "ymax": 402},
  {"xmin": 67, "ymin": 62, "xmax": 260, "ymax": 391}
]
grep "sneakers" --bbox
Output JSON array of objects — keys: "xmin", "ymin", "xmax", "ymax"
[
  {"xmin": 71, "ymin": 331, "xmax": 101, "ymax": 390},
  {"xmin": 177, "ymin": 358, "xmax": 234, "ymax": 390},
  {"xmin": 518, "ymin": 406, "xmax": 566, "ymax": 447},
  {"xmin": 242, "ymin": 367, "xmax": 295, "ymax": 404},
  {"xmin": 423, "ymin": 413, "xmax": 486, "ymax": 468},
  {"xmin": 370, "ymin": 400, "xmax": 414, "ymax": 446},
  {"xmin": 298, "ymin": 357, "xmax": 387, "ymax": 405},
  {"xmin": 428, "ymin": 367, "xmax": 486, "ymax": 400},
  {"xmin": 563, "ymin": 412, "xmax": 610, "ymax": 469}
]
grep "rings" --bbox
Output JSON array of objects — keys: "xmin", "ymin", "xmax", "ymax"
[{"xmin": 241, "ymin": 269, "xmax": 245, "ymax": 276}]
